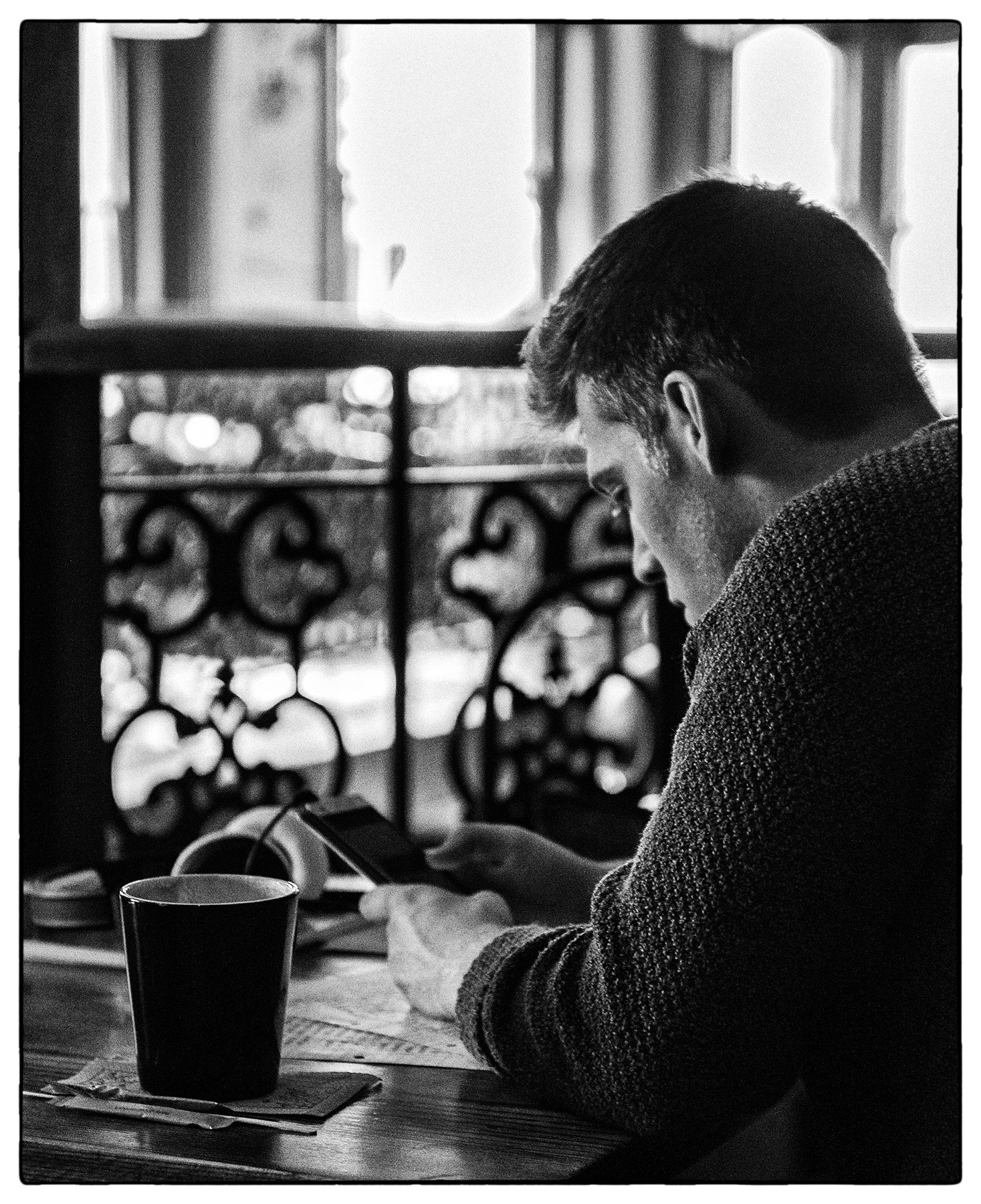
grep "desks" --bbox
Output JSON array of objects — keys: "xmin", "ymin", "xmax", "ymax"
[{"xmin": 21, "ymin": 903, "xmax": 663, "ymax": 1185}]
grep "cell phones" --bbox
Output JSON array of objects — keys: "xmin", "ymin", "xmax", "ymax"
[{"xmin": 296, "ymin": 794, "xmax": 474, "ymax": 898}]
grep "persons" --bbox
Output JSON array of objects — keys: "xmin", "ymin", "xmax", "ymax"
[{"xmin": 355, "ymin": 174, "xmax": 962, "ymax": 1180}]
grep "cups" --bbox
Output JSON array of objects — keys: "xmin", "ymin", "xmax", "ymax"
[{"xmin": 118, "ymin": 872, "xmax": 299, "ymax": 1103}]
